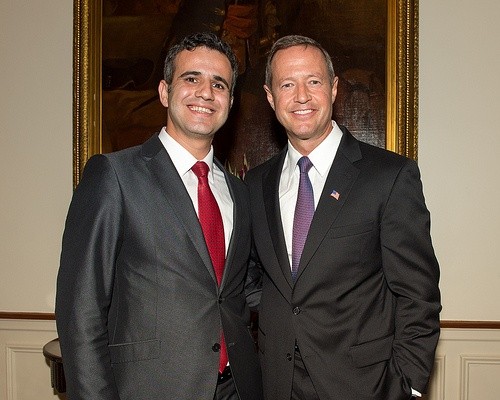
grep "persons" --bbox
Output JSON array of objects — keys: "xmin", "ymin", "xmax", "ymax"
[
  {"xmin": 54, "ymin": 31, "xmax": 261, "ymax": 400},
  {"xmin": 241, "ymin": 34, "xmax": 443, "ymax": 400}
]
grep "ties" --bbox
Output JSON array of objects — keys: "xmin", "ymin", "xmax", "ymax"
[
  {"xmin": 191, "ymin": 161, "xmax": 230, "ymax": 374},
  {"xmin": 292, "ymin": 156, "xmax": 315, "ymax": 282}
]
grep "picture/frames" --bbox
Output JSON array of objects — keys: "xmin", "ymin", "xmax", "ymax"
[{"xmin": 72, "ymin": 0, "xmax": 419, "ymax": 199}]
